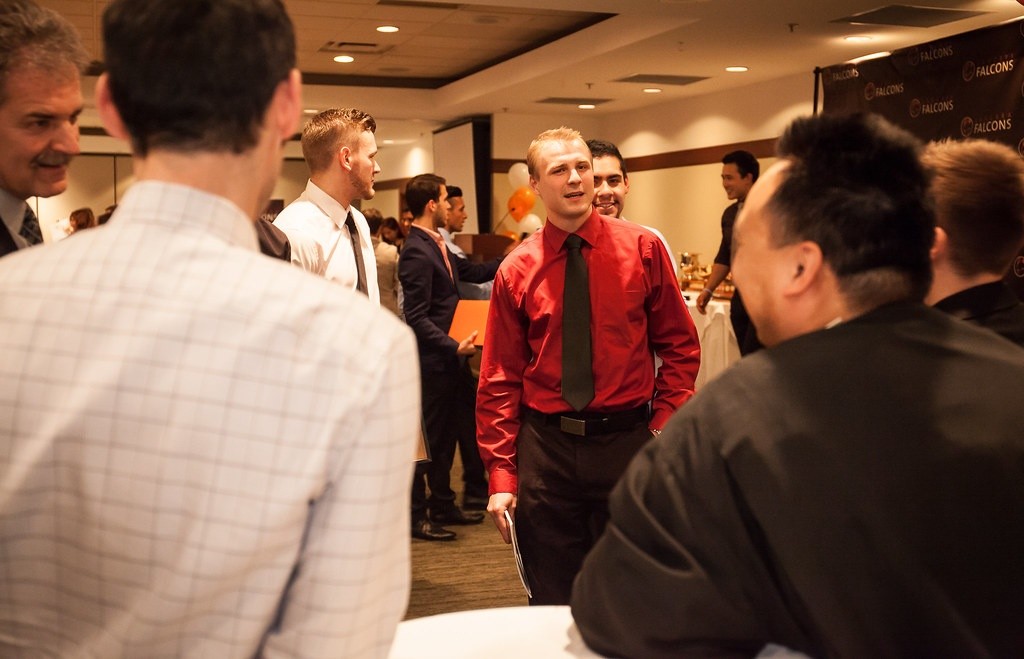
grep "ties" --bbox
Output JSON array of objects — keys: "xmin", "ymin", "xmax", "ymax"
[
  {"xmin": 18, "ymin": 205, "xmax": 43, "ymax": 246},
  {"xmin": 560, "ymin": 233, "xmax": 595, "ymax": 411},
  {"xmin": 345, "ymin": 211, "xmax": 368, "ymax": 296}
]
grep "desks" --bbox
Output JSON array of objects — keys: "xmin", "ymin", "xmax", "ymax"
[
  {"xmin": 390, "ymin": 606, "xmax": 809, "ymax": 659},
  {"xmin": 655, "ymin": 292, "xmax": 741, "ymax": 389}
]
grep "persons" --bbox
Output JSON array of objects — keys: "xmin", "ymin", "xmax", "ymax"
[
  {"xmin": 585, "ymin": 139, "xmax": 678, "ymax": 280},
  {"xmin": 397, "ymin": 173, "xmax": 478, "ymax": 542},
  {"xmin": 919, "ymin": 137, "xmax": 1024, "ymax": 352},
  {"xmin": 475, "ymin": 125, "xmax": 702, "ymax": 607},
  {"xmin": 0, "ymin": 0, "xmax": 96, "ymax": 258},
  {"xmin": 440, "ymin": 184, "xmax": 530, "ymax": 512},
  {"xmin": 568, "ymin": 105, "xmax": 1024, "ymax": 659},
  {"xmin": 696, "ymin": 150, "xmax": 760, "ymax": 358},
  {"xmin": 272, "ymin": 106, "xmax": 381, "ymax": 307},
  {"xmin": 1, "ymin": 0, "xmax": 422, "ymax": 659},
  {"xmin": 70, "ymin": 204, "xmax": 415, "ymax": 322}
]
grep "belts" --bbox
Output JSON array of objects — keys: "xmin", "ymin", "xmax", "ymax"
[{"xmin": 522, "ymin": 404, "xmax": 650, "ymax": 436}]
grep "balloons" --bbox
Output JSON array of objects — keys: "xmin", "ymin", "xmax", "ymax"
[{"xmin": 508, "ymin": 163, "xmax": 541, "ymax": 237}]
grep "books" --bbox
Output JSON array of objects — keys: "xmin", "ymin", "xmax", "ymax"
[{"xmin": 504, "ymin": 510, "xmax": 533, "ymax": 599}]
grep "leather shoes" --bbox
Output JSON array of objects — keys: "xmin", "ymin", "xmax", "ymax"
[
  {"xmin": 429, "ymin": 505, "xmax": 485, "ymax": 525},
  {"xmin": 411, "ymin": 515, "xmax": 456, "ymax": 540},
  {"xmin": 462, "ymin": 494, "xmax": 490, "ymax": 510}
]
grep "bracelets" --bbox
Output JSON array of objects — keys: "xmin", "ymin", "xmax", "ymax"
[
  {"xmin": 653, "ymin": 429, "xmax": 662, "ymax": 435},
  {"xmin": 703, "ymin": 288, "xmax": 712, "ymax": 294}
]
